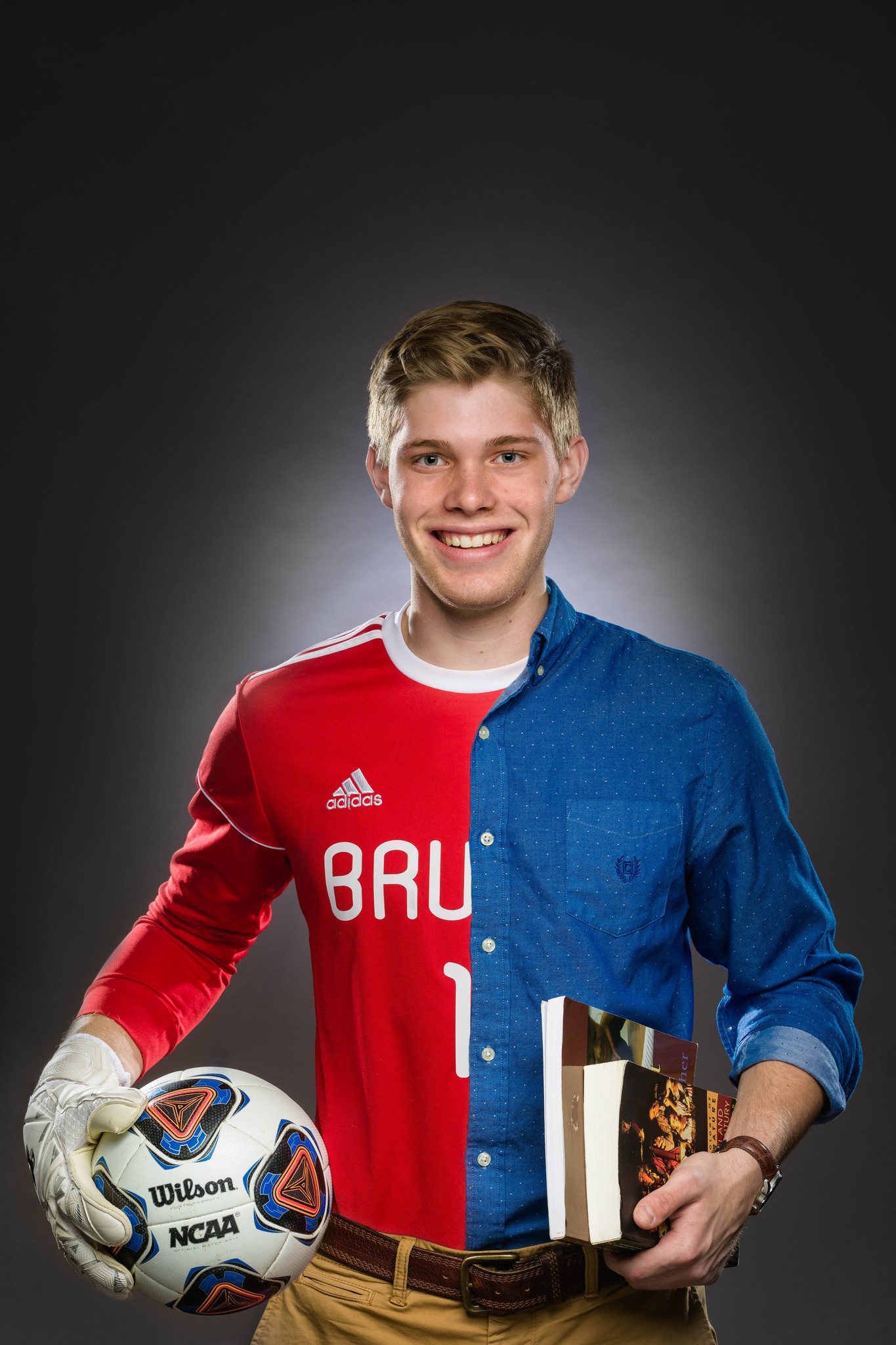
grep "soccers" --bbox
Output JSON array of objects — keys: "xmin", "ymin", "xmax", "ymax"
[{"xmin": 97, "ymin": 1064, "xmax": 334, "ymax": 1320}]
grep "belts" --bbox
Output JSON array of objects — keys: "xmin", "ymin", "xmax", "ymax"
[{"xmin": 315, "ymin": 1212, "xmax": 627, "ymax": 1318}]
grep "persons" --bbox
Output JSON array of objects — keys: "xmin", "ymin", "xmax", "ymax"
[
  {"xmin": 20, "ymin": 296, "xmax": 864, "ymax": 1345},
  {"xmin": 622, "ymin": 1068, "xmax": 694, "ymax": 1192}
]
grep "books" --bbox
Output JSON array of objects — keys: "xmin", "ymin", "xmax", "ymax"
[
  {"xmin": 540, "ymin": 995, "xmax": 700, "ymax": 1243},
  {"xmin": 584, "ymin": 1059, "xmax": 740, "ymax": 1269}
]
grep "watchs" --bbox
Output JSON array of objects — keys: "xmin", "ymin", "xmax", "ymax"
[{"xmin": 713, "ymin": 1134, "xmax": 780, "ymax": 1215}]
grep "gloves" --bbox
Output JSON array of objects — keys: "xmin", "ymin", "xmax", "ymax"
[{"xmin": 22, "ymin": 1033, "xmax": 148, "ymax": 1296}]
